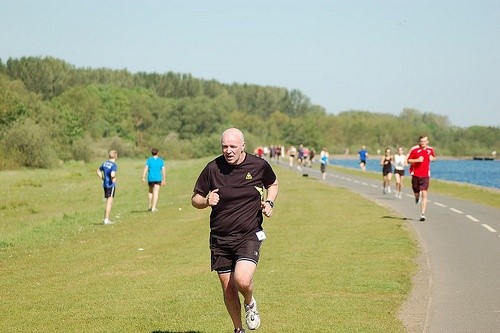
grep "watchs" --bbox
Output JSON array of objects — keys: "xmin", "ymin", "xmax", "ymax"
[{"xmin": 265, "ymin": 201, "xmax": 273, "ymax": 207}]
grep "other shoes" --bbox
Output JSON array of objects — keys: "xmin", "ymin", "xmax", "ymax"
[
  {"xmin": 384, "ymin": 188, "xmax": 386, "ymax": 194},
  {"xmin": 149, "ymin": 207, "xmax": 152, "ymax": 211},
  {"xmin": 395, "ymin": 194, "xmax": 401, "ymax": 199},
  {"xmin": 388, "ymin": 187, "xmax": 391, "ymax": 193},
  {"xmin": 151, "ymin": 208, "xmax": 157, "ymax": 211},
  {"xmin": 104, "ymin": 219, "xmax": 111, "ymax": 224},
  {"xmin": 415, "ymin": 199, "xmax": 419, "ymax": 205},
  {"xmin": 420, "ymin": 214, "xmax": 426, "ymax": 221}
]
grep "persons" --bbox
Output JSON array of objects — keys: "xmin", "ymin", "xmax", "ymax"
[
  {"xmin": 142, "ymin": 148, "xmax": 166, "ymax": 212},
  {"xmin": 379, "ymin": 136, "xmax": 436, "ymax": 221},
  {"xmin": 358, "ymin": 146, "xmax": 369, "ymax": 172},
  {"xmin": 97, "ymin": 150, "xmax": 118, "ymax": 225},
  {"xmin": 191, "ymin": 127, "xmax": 279, "ymax": 333},
  {"xmin": 254, "ymin": 143, "xmax": 331, "ymax": 182}
]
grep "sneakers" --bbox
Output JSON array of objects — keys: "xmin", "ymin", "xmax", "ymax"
[
  {"xmin": 244, "ymin": 295, "xmax": 260, "ymax": 330},
  {"xmin": 234, "ymin": 328, "xmax": 245, "ymax": 333}
]
